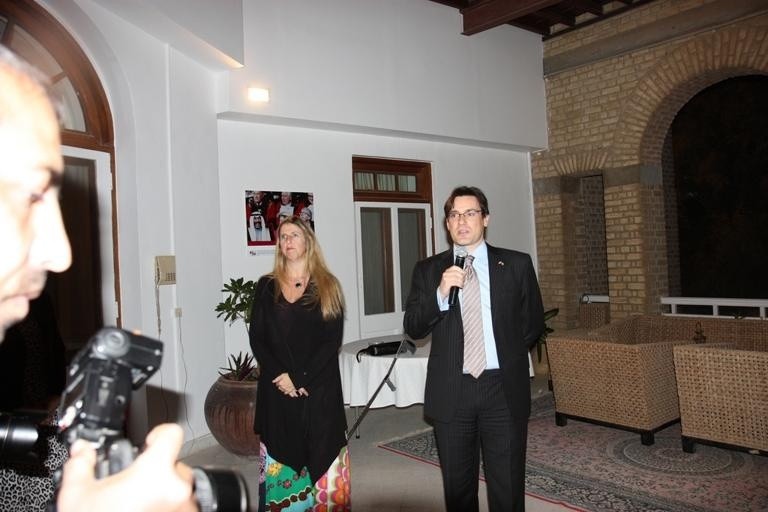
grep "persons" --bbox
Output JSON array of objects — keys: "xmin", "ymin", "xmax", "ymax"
[
  {"xmin": 0, "ymin": 43, "xmax": 201, "ymax": 512},
  {"xmin": 248, "ymin": 216, "xmax": 351, "ymax": 512},
  {"xmin": 246, "ymin": 191, "xmax": 314, "ymax": 243},
  {"xmin": 403, "ymin": 185, "xmax": 543, "ymax": 512}
]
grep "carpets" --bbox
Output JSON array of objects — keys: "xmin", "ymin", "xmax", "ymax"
[{"xmin": 378, "ymin": 391, "xmax": 767, "ymax": 512}]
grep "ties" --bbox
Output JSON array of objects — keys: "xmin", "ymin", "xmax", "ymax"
[{"xmin": 460, "ymin": 255, "xmax": 487, "ymax": 380}]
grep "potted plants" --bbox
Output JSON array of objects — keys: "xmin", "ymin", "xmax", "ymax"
[{"xmin": 203, "ymin": 278, "xmax": 260, "ymax": 456}]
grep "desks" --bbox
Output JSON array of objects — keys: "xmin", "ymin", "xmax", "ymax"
[{"xmin": 341, "ymin": 335, "xmax": 535, "ymax": 440}]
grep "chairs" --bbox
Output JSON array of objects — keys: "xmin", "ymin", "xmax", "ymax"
[
  {"xmin": 673, "ymin": 342, "xmax": 767, "ymax": 453},
  {"xmin": 546, "ymin": 334, "xmax": 697, "ymax": 445}
]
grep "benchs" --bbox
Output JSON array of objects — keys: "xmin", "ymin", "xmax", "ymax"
[{"xmin": 588, "ymin": 315, "xmax": 768, "ymax": 352}]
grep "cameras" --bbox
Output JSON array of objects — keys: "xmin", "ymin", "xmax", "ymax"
[{"xmin": 0, "ymin": 323, "xmax": 253, "ymax": 512}]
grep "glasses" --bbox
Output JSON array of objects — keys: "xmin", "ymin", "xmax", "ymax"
[
  {"xmin": 448, "ymin": 209, "xmax": 483, "ymax": 218},
  {"xmin": 298, "ymin": 212, "xmax": 310, "ymax": 216}
]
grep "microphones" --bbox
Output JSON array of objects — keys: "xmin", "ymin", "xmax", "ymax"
[{"xmin": 448, "ymin": 245, "xmax": 470, "ymax": 304}]
